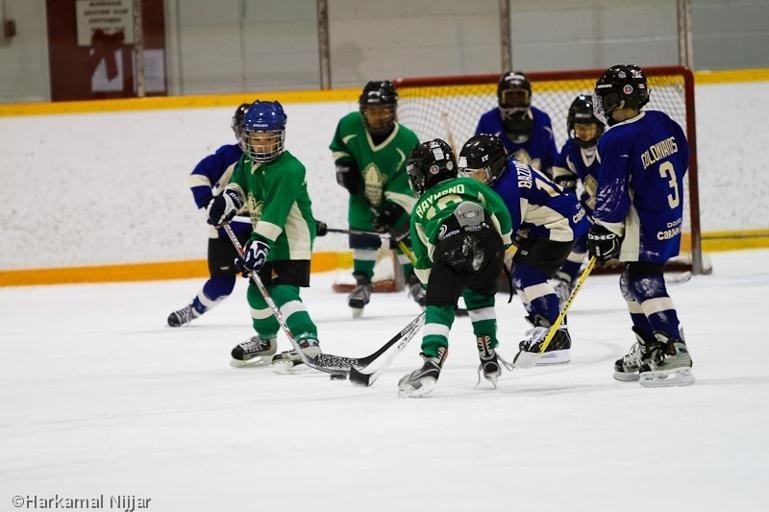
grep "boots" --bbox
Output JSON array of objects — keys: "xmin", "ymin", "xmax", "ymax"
[
  {"xmin": 472, "ymin": 332, "xmax": 510, "ymax": 392},
  {"xmin": 273, "ymin": 335, "xmax": 324, "ymax": 376},
  {"xmin": 228, "ymin": 334, "xmax": 278, "ymax": 370},
  {"xmin": 345, "ymin": 268, "xmax": 372, "ymax": 319},
  {"xmin": 545, "ymin": 270, "xmax": 575, "ymax": 304},
  {"xmin": 638, "ymin": 331, "xmax": 696, "ymax": 387},
  {"xmin": 403, "ymin": 269, "xmax": 428, "ymax": 308},
  {"xmin": 168, "ymin": 300, "xmax": 206, "ymax": 329},
  {"xmin": 614, "ymin": 326, "xmax": 655, "ymax": 381},
  {"xmin": 396, "ymin": 346, "xmax": 445, "ymax": 397},
  {"xmin": 517, "ymin": 313, "xmax": 570, "ymax": 367}
]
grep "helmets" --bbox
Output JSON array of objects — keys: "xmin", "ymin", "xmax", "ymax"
[
  {"xmin": 241, "ymin": 100, "xmax": 288, "ymax": 162},
  {"xmin": 406, "ymin": 136, "xmax": 455, "ymax": 191},
  {"xmin": 498, "ymin": 72, "xmax": 532, "ymax": 117},
  {"xmin": 567, "ymin": 94, "xmax": 608, "ymax": 147},
  {"xmin": 458, "ymin": 133, "xmax": 509, "ymax": 185},
  {"xmin": 594, "ymin": 63, "xmax": 651, "ymax": 115},
  {"xmin": 233, "ymin": 103, "xmax": 252, "ymax": 153},
  {"xmin": 359, "ymin": 79, "xmax": 398, "ymax": 133}
]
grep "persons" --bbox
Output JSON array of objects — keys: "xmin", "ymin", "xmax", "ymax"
[
  {"xmin": 397, "ymin": 138, "xmax": 514, "ymax": 401},
  {"xmin": 207, "ymin": 99, "xmax": 321, "ymax": 373},
  {"xmin": 167, "ymin": 102, "xmax": 326, "ymax": 328},
  {"xmin": 582, "ymin": 63, "xmax": 695, "ymax": 388},
  {"xmin": 457, "ymin": 132, "xmax": 595, "ymax": 366},
  {"xmin": 473, "ymin": 69, "xmax": 557, "ymax": 172},
  {"xmin": 551, "ymin": 94, "xmax": 610, "ymax": 300},
  {"xmin": 328, "ymin": 80, "xmax": 425, "ymax": 320}
]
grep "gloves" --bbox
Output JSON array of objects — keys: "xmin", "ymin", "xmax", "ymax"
[
  {"xmin": 334, "ymin": 163, "xmax": 366, "ymax": 196},
  {"xmin": 233, "ymin": 239, "xmax": 270, "ymax": 280},
  {"xmin": 377, "ymin": 203, "xmax": 403, "ymax": 235},
  {"xmin": 586, "ymin": 224, "xmax": 620, "ymax": 269},
  {"xmin": 207, "ymin": 187, "xmax": 244, "ymax": 228}
]
